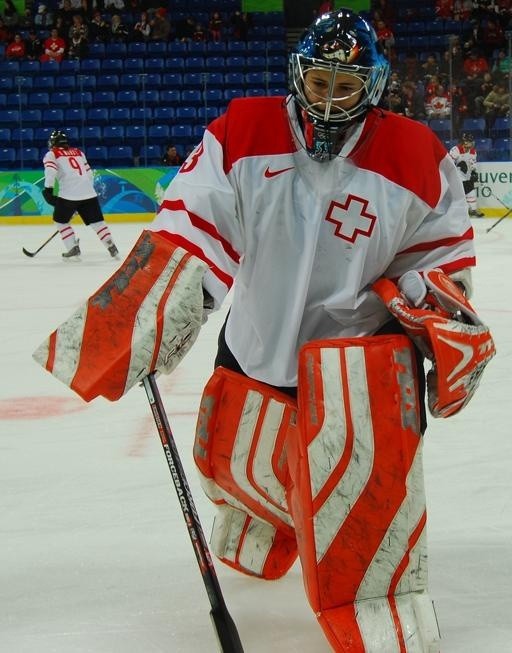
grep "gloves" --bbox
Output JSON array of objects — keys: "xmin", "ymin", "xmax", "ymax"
[
  {"xmin": 456, "ymin": 160, "xmax": 467, "ymax": 174},
  {"xmin": 469, "ymin": 170, "xmax": 478, "ymax": 183},
  {"xmin": 41, "ymin": 188, "xmax": 60, "ymax": 207}
]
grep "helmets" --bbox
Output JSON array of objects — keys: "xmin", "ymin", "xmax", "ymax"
[
  {"xmin": 289, "ymin": 8, "xmax": 390, "ymax": 129},
  {"xmin": 46, "ymin": 130, "xmax": 69, "ymax": 150},
  {"xmin": 461, "ymin": 132, "xmax": 475, "ymax": 147}
]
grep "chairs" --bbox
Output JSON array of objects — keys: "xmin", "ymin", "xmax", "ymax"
[{"xmin": 0, "ymin": 12, "xmax": 512, "ymax": 165}]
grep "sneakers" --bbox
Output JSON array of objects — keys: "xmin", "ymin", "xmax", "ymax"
[
  {"xmin": 62, "ymin": 245, "xmax": 80, "ymax": 257},
  {"xmin": 107, "ymin": 245, "xmax": 118, "ymax": 257},
  {"xmin": 469, "ymin": 208, "xmax": 484, "ymax": 217}
]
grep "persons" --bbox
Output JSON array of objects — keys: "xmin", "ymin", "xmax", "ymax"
[
  {"xmin": 449, "ymin": 133, "xmax": 485, "ymax": 217},
  {"xmin": 42, "ymin": 129, "xmax": 120, "ymax": 258},
  {"xmin": 32, "ymin": 8, "xmax": 497, "ymax": 653},
  {"xmin": 0, "ymin": 0, "xmax": 251, "ymax": 63},
  {"xmin": 160, "ymin": 144, "xmax": 183, "ymax": 166},
  {"xmin": 319, "ymin": 0, "xmax": 512, "ymax": 120}
]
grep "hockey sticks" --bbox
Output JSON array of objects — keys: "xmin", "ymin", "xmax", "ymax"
[
  {"xmin": 474, "ymin": 210, "xmax": 512, "ymax": 235},
  {"xmin": 23, "ymin": 230, "xmax": 59, "ymax": 257}
]
